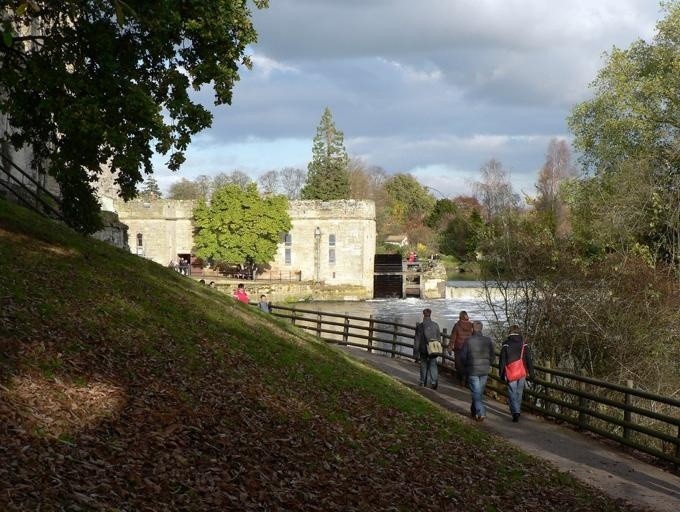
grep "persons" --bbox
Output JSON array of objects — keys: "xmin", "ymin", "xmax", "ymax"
[
  {"xmin": 499, "ymin": 325, "xmax": 534, "ymax": 423},
  {"xmin": 200, "ymin": 279, "xmax": 215, "ymax": 289},
  {"xmin": 258, "ymin": 295, "xmax": 269, "ymax": 314},
  {"xmin": 179, "ymin": 257, "xmax": 188, "ymax": 275},
  {"xmin": 461, "ymin": 321, "xmax": 496, "ymax": 421},
  {"xmin": 448, "ymin": 311, "xmax": 473, "ymax": 386},
  {"xmin": 409, "ymin": 252, "xmax": 418, "ymax": 262},
  {"xmin": 413, "ymin": 309, "xmax": 441, "ymax": 390},
  {"xmin": 234, "ymin": 284, "xmax": 248, "ymax": 305}
]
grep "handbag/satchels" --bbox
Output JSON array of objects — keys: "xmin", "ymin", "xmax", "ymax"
[
  {"xmin": 425, "ymin": 339, "xmax": 443, "ymax": 355},
  {"xmin": 504, "ymin": 358, "xmax": 526, "ymax": 381}
]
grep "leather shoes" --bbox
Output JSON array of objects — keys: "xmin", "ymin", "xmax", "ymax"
[
  {"xmin": 418, "ymin": 384, "xmax": 437, "ymax": 389},
  {"xmin": 512, "ymin": 413, "xmax": 520, "ymax": 422}
]
